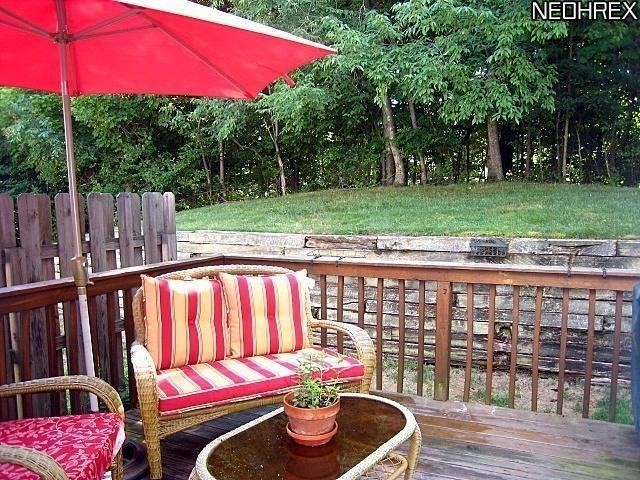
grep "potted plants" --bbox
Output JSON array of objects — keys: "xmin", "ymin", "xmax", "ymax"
[{"xmin": 282, "ymin": 349, "xmax": 351, "ymax": 447}]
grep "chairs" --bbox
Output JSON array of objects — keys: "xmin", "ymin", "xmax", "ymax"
[{"xmin": 0, "ymin": 374, "xmax": 126, "ymax": 479}]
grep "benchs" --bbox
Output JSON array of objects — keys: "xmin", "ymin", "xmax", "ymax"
[{"xmin": 130, "ymin": 264, "xmax": 378, "ymax": 478}]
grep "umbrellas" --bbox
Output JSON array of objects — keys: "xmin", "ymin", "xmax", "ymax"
[{"xmin": 1, "ymin": 1, "xmax": 345, "ymax": 412}]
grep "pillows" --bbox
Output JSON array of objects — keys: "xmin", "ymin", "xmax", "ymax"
[{"xmin": 140, "ymin": 272, "xmax": 311, "ymax": 369}]
